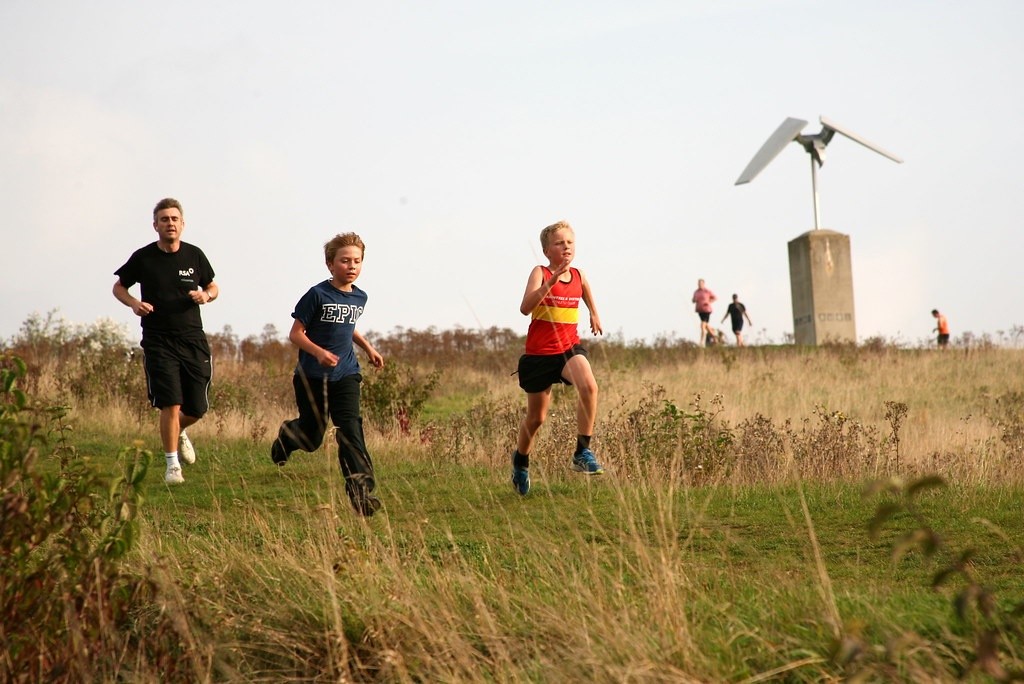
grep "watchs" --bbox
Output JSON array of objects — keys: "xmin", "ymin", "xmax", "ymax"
[{"xmin": 205, "ymin": 289, "xmax": 213, "ymax": 303}]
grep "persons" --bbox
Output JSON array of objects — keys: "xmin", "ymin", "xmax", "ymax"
[
  {"xmin": 929, "ymin": 306, "xmax": 951, "ymax": 348},
  {"xmin": 692, "ymin": 278, "xmax": 721, "ymax": 347},
  {"xmin": 113, "ymin": 194, "xmax": 221, "ymax": 487},
  {"xmin": 722, "ymin": 292, "xmax": 753, "ymax": 346},
  {"xmin": 706, "ymin": 325, "xmax": 727, "ymax": 348},
  {"xmin": 507, "ymin": 221, "xmax": 606, "ymax": 496},
  {"xmin": 267, "ymin": 229, "xmax": 390, "ymax": 516}
]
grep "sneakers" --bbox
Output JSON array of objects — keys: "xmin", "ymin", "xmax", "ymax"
[
  {"xmin": 510, "ymin": 450, "xmax": 530, "ymax": 495},
  {"xmin": 271, "ymin": 420, "xmax": 293, "ymax": 466},
  {"xmin": 569, "ymin": 448, "xmax": 604, "ymax": 474},
  {"xmin": 356, "ymin": 497, "xmax": 381, "ymax": 517},
  {"xmin": 165, "ymin": 462, "xmax": 184, "ymax": 486},
  {"xmin": 177, "ymin": 428, "xmax": 196, "ymax": 465}
]
